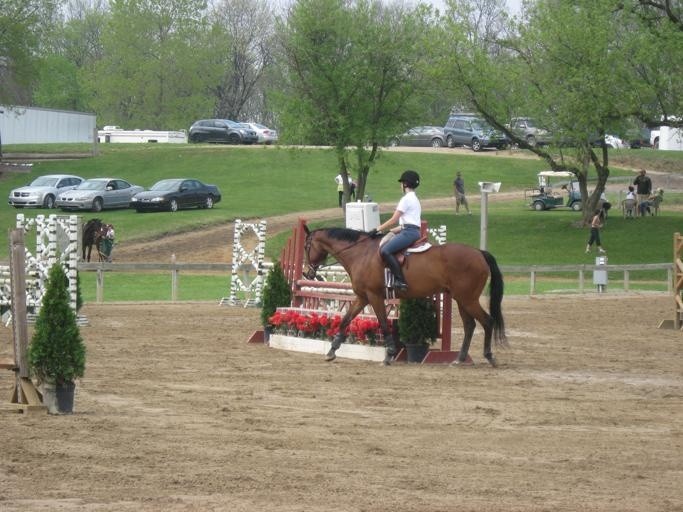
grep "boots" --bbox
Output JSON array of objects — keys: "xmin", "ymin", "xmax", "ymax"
[{"xmin": 383, "ymin": 254, "xmax": 407, "ymax": 287}]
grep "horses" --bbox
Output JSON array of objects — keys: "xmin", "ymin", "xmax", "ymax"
[
  {"xmin": 303, "ymin": 223, "xmax": 508, "ymax": 367},
  {"xmin": 82, "ymin": 218, "xmax": 103, "ymax": 263}
]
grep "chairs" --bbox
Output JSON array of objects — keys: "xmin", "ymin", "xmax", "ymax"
[{"xmin": 622, "ymin": 199, "xmax": 638, "ymax": 219}]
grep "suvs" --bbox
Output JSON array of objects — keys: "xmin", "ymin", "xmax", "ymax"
[{"xmin": 187, "ymin": 112, "xmax": 279, "ymax": 145}]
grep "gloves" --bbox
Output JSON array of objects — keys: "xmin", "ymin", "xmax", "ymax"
[{"xmin": 368, "ymin": 229, "xmax": 377, "ymax": 236}]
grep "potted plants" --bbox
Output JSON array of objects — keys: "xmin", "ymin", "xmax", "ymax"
[
  {"xmin": 261, "ymin": 260, "xmax": 292, "ymax": 344},
  {"xmin": 27, "ymin": 261, "xmax": 85, "ymax": 413}
]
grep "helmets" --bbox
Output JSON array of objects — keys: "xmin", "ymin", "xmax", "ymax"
[{"xmin": 398, "ymin": 170, "xmax": 419, "ymax": 188}]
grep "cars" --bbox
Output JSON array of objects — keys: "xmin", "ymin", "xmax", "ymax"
[
  {"xmin": 379, "ymin": 105, "xmax": 662, "ymax": 152},
  {"xmin": 8, "ymin": 175, "xmax": 221, "ymax": 212}
]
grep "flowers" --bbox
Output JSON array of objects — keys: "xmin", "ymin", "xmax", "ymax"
[{"xmin": 266, "ymin": 311, "xmax": 388, "ymax": 345}]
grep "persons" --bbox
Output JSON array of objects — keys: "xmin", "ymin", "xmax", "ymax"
[
  {"xmin": 453, "ymin": 171, "xmax": 473, "ymax": 216},
  {"xmin": 625, "ymin": 185, "xmax": 637, "ymax": 219},
  {"xmin": 368, "ymin": 170, "xmax": 421, "ymax": 290},
  {"xmin": 638, "ymin": 188, "xmax": 663, "ymax": 217},
  {"xmin": 633, "ymin": 169, "xmax": 652, "ymax": 216},
  {"xmin": 105, "ymin": 224, "xmax": 115, "ymax": 263},
  {"xmin": 585, "ymin": 210, "xmax": 607, "ymax": 256},
  {"xmin": 335, "ymin": 170, "xmax": 357, "ymax": 208}
]
